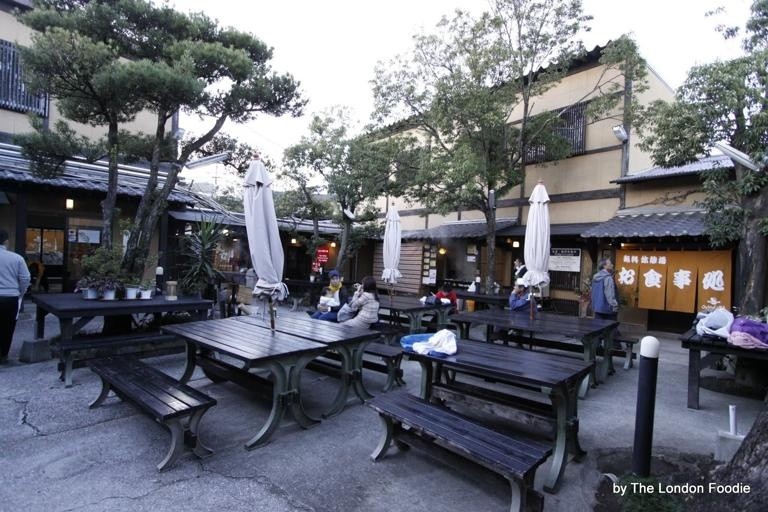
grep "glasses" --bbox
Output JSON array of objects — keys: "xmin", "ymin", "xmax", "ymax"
[
  {"xmin": 186, "ymin": 152, "xmax": 231, "ymax": 170},
  {"xmin": 344, "ymin": 209, "xmax": 355, "ymax": 220},
  {"xmin": 613, "ymin": 125, "xmax": 628, "ymax": 144}
]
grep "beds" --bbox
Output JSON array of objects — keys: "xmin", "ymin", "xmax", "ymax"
[
  {"xmin": 525, "ymin": 179, "xmax": 551, "ymax": 320},
  {"xmin": 382, "ymin": 203, "xmax": 402, "ymax": 309},
  {"xmin": 243, "ymin": 155, "xmax": 291, "ymax": 330}
]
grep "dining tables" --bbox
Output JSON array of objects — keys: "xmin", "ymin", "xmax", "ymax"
[
  {"xmin": 181, "ymin": 219, "xmax": 220, "ymax": 296},
  {"xmin": 69, "ymin": 251, "xmax": 168, "ymax": 300}
]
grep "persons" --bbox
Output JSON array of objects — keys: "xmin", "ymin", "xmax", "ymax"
[
  {"xmin": 311, "ymin": 270, "xmax": 349, "ymax": 321},
  {"xmin": 339, "ymin": 275, "xmax": 380, "ymax": 329},
  {"xmin": 0, "ymin": 230, "xmax": 30, "ymax": 363},
  {"xmin": 514, "ymin": 258, "xmax": 527, "ymax": 279},
  {"xmin": 495, "ymin": 278, "xmax": 537, "ymax": 332},
  {"xmin": 592, "ymin": 258, "xmax": 619, "ymax": 372},
  {"xmin": 320, "ymin": 287, "xmax": 340, "ymax": 314}
]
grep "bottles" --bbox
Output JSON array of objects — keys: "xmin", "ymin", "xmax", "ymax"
[{"xmin": 329, "ymin": 270, "xmax": 339, "ymax": 278}]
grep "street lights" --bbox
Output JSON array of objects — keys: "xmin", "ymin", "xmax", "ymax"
[{"xmin": 337, "ymin": 302, "xmax": 358, "ymax": 322}]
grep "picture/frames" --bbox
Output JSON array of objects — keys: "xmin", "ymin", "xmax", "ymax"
[
  {"xmin": 28, "ymin": 293, "xmax": 214, "ymax": 389},
  {"xmin": 163, "ymin": 313, "xmax": 336, "ymax": 449},
  {"xmin": 235, "ymin": 297, "xmax": 370, "ymax": 419},
  {"xmin": 397, "ymin": 335, "xmax": 589, "ymax": 494},
  {"xmin": 680, "ymin": 324, "xmax": 767, "ymax": 413},
  {"xmin": 363, "ymin": 291, "xmax": 640, "ymax": 398}
]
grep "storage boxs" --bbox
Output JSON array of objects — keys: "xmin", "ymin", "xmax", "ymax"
[
  {"xmin": 362, "ymin": 381, "xmax": 556, "ymax": 510},
  {"xmin": 86, "ymin": 357, "xmax": 216, "ymax": 463}
]
